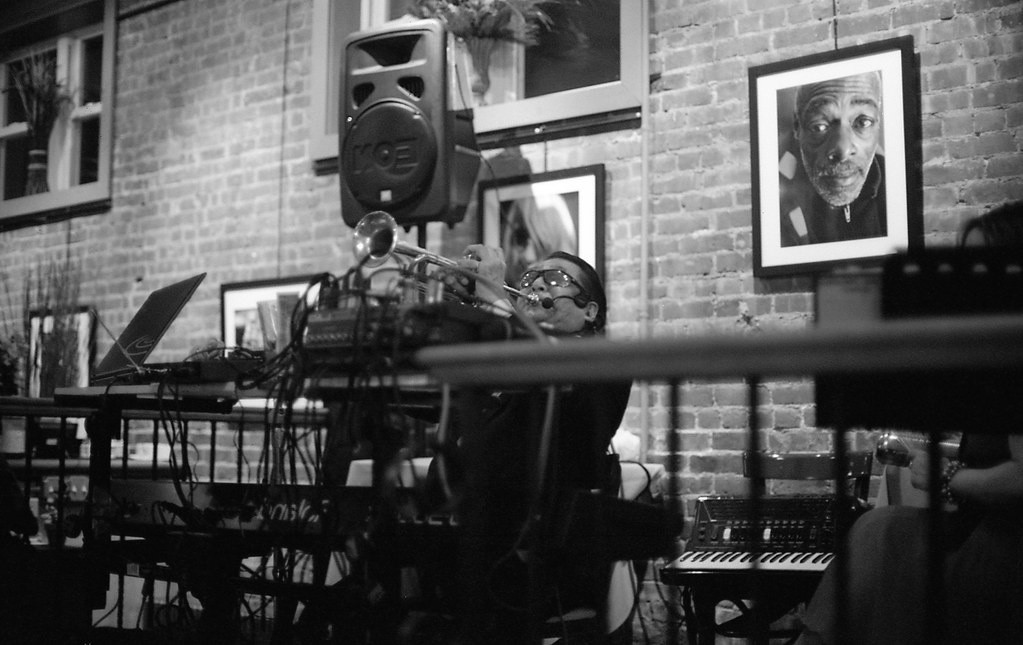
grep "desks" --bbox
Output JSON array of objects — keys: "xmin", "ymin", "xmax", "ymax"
[
  {"xmin": 416, "ymin": 319, "xmax": 1023, "ymax": 645},
  {"xmin": 53, "ymin": 371, "xmax": 424, "ymax": 645}
]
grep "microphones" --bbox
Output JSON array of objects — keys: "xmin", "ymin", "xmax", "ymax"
[{"xmin": 542, "ymin": 294, "xmax": 588, "ymax": 310}]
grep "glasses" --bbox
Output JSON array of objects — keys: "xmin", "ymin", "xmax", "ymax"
[{"xmin": 520, "ymin": 270, "xmax": 587, "ymax": 294}]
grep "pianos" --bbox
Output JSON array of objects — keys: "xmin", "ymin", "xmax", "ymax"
[{"xmin": 659, "ymin": 491, "xmax": 873, "ymax": 586}]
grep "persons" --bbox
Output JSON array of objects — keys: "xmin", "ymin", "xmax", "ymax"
[
  {"xmin": 422, "ymin": 241, "xmax": 633, "ymax": 645},
  {"xmin": 499, "ymin": 193, "xmax": 576, "ymax": 297},
  {"xmin": 782, "ymin": 67, "xmax": 888, "ymax": 247}
]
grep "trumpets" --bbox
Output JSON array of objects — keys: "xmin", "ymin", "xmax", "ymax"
[{"xmin": 351, "ymin": 209, "xmax": 540, "ymax": 317}]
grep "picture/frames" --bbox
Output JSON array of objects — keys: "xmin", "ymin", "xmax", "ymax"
[
  {"xmin": 22, "ymin": 305, "xmax": 98, "ymax": 443},
  {"xmin": 748, "ymin": 35, "xmax": 924, "ymax": 279},
  {"xmin": 476, "ymin": 162, "xmax": 607, "ymax": 315},
  {"xmin": 216, "ymin": 273, "xmax": 336, "ymax": 396}
]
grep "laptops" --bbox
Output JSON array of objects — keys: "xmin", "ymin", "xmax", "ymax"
[{"xmin": 91, "ymin": 272, "xmax": 264, "ymax": 385}]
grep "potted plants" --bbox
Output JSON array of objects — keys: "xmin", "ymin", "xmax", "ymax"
[
  {"xmin": 408, "ymin": 0, "xmax": 588, "ymax": 109},
  {"xmin": 4, "ymin": 51, "xmax": 76, "ymax": 195}
]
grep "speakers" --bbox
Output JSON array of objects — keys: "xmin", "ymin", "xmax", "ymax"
[{"xmin": 339, "ymin": 18, "xmax": 481, "ymax": 234}]
